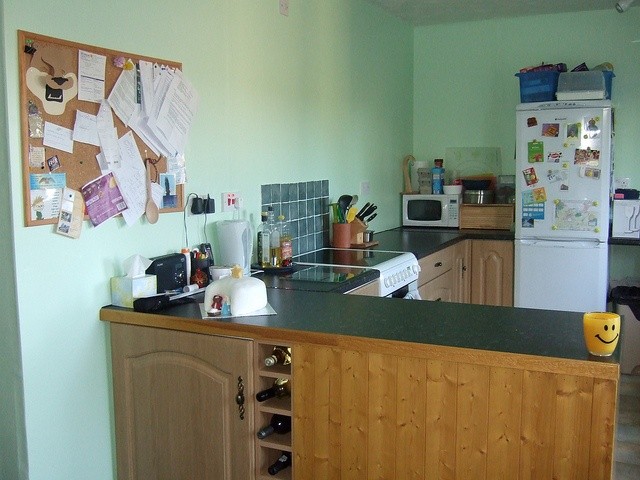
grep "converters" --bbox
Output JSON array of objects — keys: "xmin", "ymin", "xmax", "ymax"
[
  {"xmin": 203, "ymin": 194, "xmax": 215, "ymax": 213},
  {"xmin": 191, "ymin": 198, "xmax": 203, "ymax": 214}
]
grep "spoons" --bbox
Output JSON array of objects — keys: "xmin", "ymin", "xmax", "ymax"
[{"xmin": 337, "ymin": 195, "xmax": 353, "ymax": 224}]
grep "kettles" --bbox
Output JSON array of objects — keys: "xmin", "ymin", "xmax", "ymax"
[{"xmin": 216, "ymin": 220, "xmax": 253, "ymax": 277}]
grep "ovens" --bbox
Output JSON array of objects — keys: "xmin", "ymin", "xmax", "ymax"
[{"xmin": 292, "ymin": 247, "xmax": 422, "ymax": 301}]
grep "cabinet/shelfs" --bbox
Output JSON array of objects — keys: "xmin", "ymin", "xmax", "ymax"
[
  {"xmin": 467, "ymin": 236, "xmax": 513, "ymax": 309},
  {"xmin": 416, "ymin": 240, "xmax": 466, "ymax": 305},
  {"xmin": 106, "ymin": 323, "xmax": 254, "ymax": 478},
  {"xmin": 253, "ymin": 341, "xmax": 293, "ymax": 480}
]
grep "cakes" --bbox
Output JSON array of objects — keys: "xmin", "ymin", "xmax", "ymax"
[{"xmin": 203, "ymin": 265, "xmax": 267, "ymax": 315}]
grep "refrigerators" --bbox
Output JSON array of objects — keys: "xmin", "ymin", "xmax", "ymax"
[{"xmin": 515, "ymin": 100, "xmax": 611, "ymax": 314}]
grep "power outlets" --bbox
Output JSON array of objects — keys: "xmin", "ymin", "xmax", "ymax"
[
  {"xmin": 186, "ymin": 194, "xmax": 203, "ymax": 217},
  {"xmin": 235, "ymin": 194, "xmax": 241, "ymax": 212},
  {"xmin": 203, "ymin": 194, "xmax": 216, "ymax": 215}
]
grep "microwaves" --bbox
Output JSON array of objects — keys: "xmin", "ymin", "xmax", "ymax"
[{"xmin": 402, "ymin": 195, "xmax": 459, "ymax": 227}]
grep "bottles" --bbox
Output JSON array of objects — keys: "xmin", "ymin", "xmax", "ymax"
[
  {"xmin": 268, "ymin": 451, "xmax": 291, "ymax": 475},
  {"xmin": 264, "ymin": 345, "xmax": 291, "ymax": 367},
  {"xmin": 267, "ymin": 207, "xmax": 281, "ymax": 269},
  {"xmin": 277, "ymin": 216, "xmax": 293, "ymax": 268},
  {"xmin": 432, "ymin": 158, "xmax": 445, "ymax": 195},
  {"xmin": 256, "ymin": 378, "xmax": 291, "ymax": 402},
  {"xmin": 257, "ymin": 414, "xmax": 291, "ymax": 439},
  {"xmin": 257, "ymin": 212, "xmax": 273, "ymax": 268}
]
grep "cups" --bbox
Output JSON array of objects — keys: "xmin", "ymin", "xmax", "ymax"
[{"xmin": 583, "ymin": 313, "xmax": 621, "ymax": 357}]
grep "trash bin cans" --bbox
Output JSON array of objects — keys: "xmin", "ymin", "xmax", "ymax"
[{"xmin": 610, "ymin": 286, "xmax": 640, "ymax": 375}]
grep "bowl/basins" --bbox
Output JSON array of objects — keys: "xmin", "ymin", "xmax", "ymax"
[
  {"xmin": 461, "ymin": 180, "xmax": 492, "ymax": 190},
  {"xmin": 442, "ymin": 185, "xmax": 463, "ymax": 195}
]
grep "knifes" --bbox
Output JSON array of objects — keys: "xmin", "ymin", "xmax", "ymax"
[
  {"xmin": 355, "ymin": 202, "xmax": 370, "ymax": 218},
  {"xmin": 360, "ymin": 206, "xmax": 377, "ymax": 221},
  {"xmin": 364, "ymin": 213, "xmax": 377, "ymax": 226},
  {"xmin": 359, "ymin": 204, "xmax": 374, "ymax": 219}
]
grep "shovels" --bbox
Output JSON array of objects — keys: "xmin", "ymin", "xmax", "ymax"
[{"xmin": 346, "ymin": 208, "xmax": 360, "ymax": 223}]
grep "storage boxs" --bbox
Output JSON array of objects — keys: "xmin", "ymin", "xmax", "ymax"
[
  {"xmin": 515, "ymin": 71, "xmax": 559, "ymax": 103},
  {"xmin": 603, "ymin": 71, "xmax": 616, "ymax": 99},
  {"xmin": 459, "ymin": 203, "xmax": 514, "ymax": 232},
  {"xmin": 555, "ymin": 71, "xmax": 605, "ymax": 100}
]
